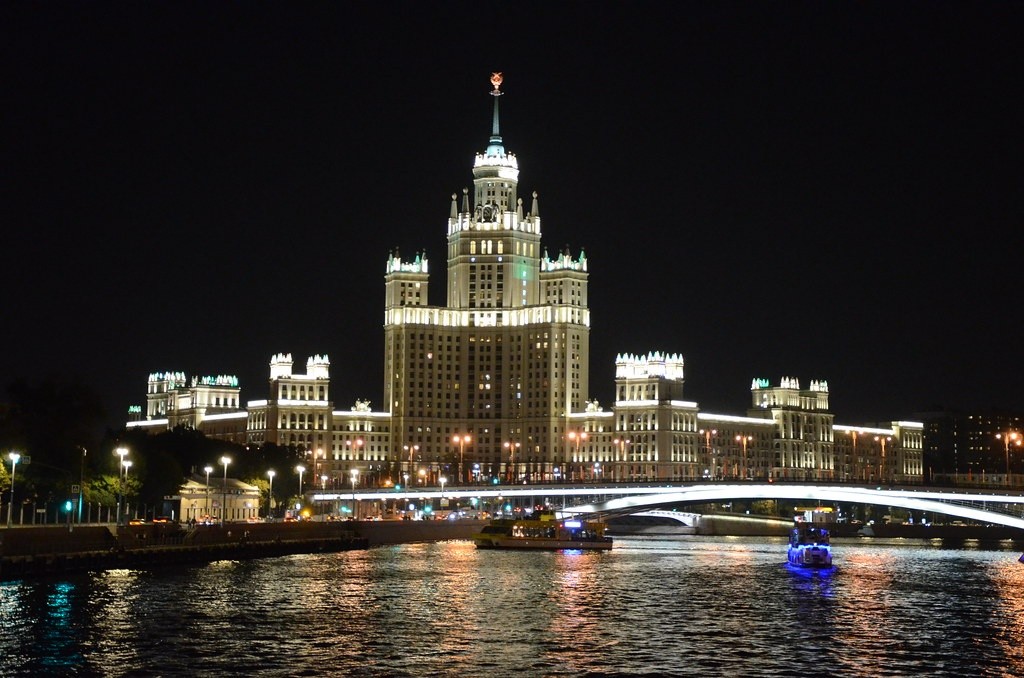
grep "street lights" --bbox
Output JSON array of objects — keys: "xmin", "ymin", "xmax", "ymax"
[
  {"xmin": 350, "ymin": 477, "xmax": 360, "ymax": 520},
  {"xmin": 116, "ymin": 448, "xmax": 129, "ymax": 524},
  {"xmin": 307, "ymin": 448, "xmax": 323, "ymax": 485},
  {"xmin": 404, "ymin": 444, "xmax": 420, "ymax": 485},
  {"xmin": 267, "ymin": 470, "xmax": 276, "ymax": 515},
  {"xmin": 219, "ymin": 456, "xmax": 232, "ymax": 523},
  {"xmin": 204, "ymin": 466, "xmax": 214, "ymax": 514},
  {"xmin": 298, "ymin": 465, "xmax": 306, "ymax": 497},
  {"xmin": 736, "ymin": 434, "xmax": 754, "ymax": 479},
  {"xmin": 845, "ymin": 430, "xmax": 863, "ymax": 448},
  {"xmin": 320, "ymin": 475, "xmax": 330, "ymax": 522},
  {"xmin": 700, "ymin": 429, "xmax": 716, "ymax": 466},
  {"xmin": 996, "ymin": 430, "xmax": 1021, "ymax": 476},
  {"xmin": 613, "ymin": 439, "xmax": 631, "ymax": 461},
  {"xmin": 7, "ymin": 453, "xmax": 21, "ymax": 527},
  {"xmin": 874, "ymin": 435, "xmax": 894, "ymax": 457},
  {"xmin": 570, "ymin": 433, "xmax": 587, "ymax": 461},
  {"xmin": 121, "ymin": 460, "xmax": 133, "ymax": 513},
  {"xmin": 504, "ymin": 441, "xmax": 520, "ymax": 483},
  {"xmin": 455, "ymin": 434, "xmax": 470, "ymax": 463}
]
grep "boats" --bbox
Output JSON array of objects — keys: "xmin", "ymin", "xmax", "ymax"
[
  {"xmin": 786, "ymin": 523, "xmax": 834, "ymax": 569},
  {"xmin": 472, "ymin": 507, "xmax": 613, "ymax": 551}
]
